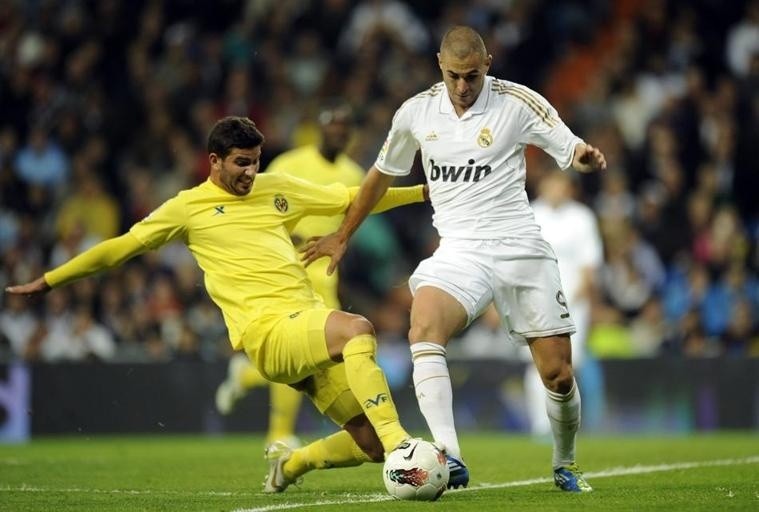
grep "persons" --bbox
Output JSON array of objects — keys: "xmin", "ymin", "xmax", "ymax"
[
  {"xmin": 214, "ymin": 100, "xmax": 369, "ymax": 461},
  {"xmin": 4, "ymin": 110, "xmax": 432, "ymax": 499},
  {"xmin": 0, "ymin": 1, "xmax": 758, "ymax": 363},
  {"xmin": 297, "ymin": 21, "xmax": 612, "ymax": 495},
  {"xmin": 510, "ymin": 165, "xmax": 609, "ymax": 444}
]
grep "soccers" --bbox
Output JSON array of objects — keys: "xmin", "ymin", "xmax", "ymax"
[{"xmin": 383, "ymin": 439, "xmax": 450, "ymax": 502}]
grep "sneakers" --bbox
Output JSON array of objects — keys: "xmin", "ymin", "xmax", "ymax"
[
  {"xmin": 262, "ymin": 441, "xmax": 304, "ymax": 496},
  {"xmin": 382, "ymin": 438, "xmax": 472, "ymax": 488},
  {"xmin": 551, "ymin": 465, "xmax": 594, "ymax": 493},
  {"xmin": 214, "ymin": 350, "xmax": 253, "ymax": 417}
]
